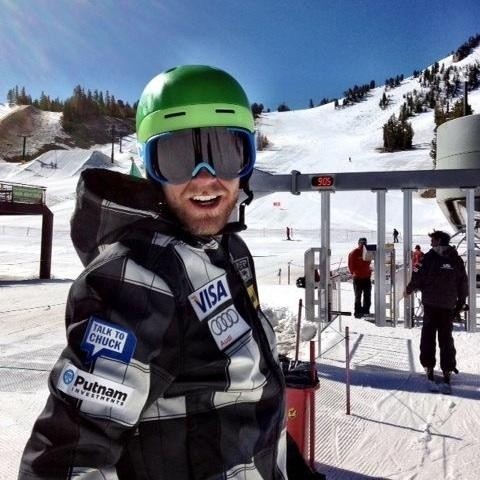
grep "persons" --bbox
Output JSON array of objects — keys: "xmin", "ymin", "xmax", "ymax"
[
  {"xmin": 286, "ymin": 227, "xmax": 290, "ymax": 240},
  {"xmin": 348, "ymin": 238, "xmax": 372, "ymax": 318},
  {"xmin": 412, "ymin": 245, "xmax": 424, "ymax": 278},
  {"xmin": 392, "ymin": 229, "xmax": 398, "ymax": 243},
  {"xmin": 402, "ymin": 229, "xmax": 469, "ymax": 394},
  {"xmin": 18, "ymin": 65, "xmax": 318, "ymax": 480}
]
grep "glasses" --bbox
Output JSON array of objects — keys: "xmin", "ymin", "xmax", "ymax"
[{"xmin": 140, "ymin": 128, "xmax": 258, "ymax": 185}]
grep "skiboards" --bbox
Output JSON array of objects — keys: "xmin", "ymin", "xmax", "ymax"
[{"xmin": 428, "ymin": 380, "xmax": 452, "ymax": 394}]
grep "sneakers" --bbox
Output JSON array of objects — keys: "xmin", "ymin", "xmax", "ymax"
[
  {"xmin": 442, "ymin": 369, "xmax": 452, "ymax": 382},
  {"xmin": 424, "ymin": 366, "xmax": 434, "ymax": 380}
]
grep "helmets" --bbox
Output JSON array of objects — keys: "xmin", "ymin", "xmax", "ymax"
[{"xmin": 134, "ymin": 63, "xmax": 256, "ymax": 150}]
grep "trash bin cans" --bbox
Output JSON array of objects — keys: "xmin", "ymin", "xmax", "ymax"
[{"xmin": 278, "ymin": 361, "xmax": 320, "ymax": 471}]
